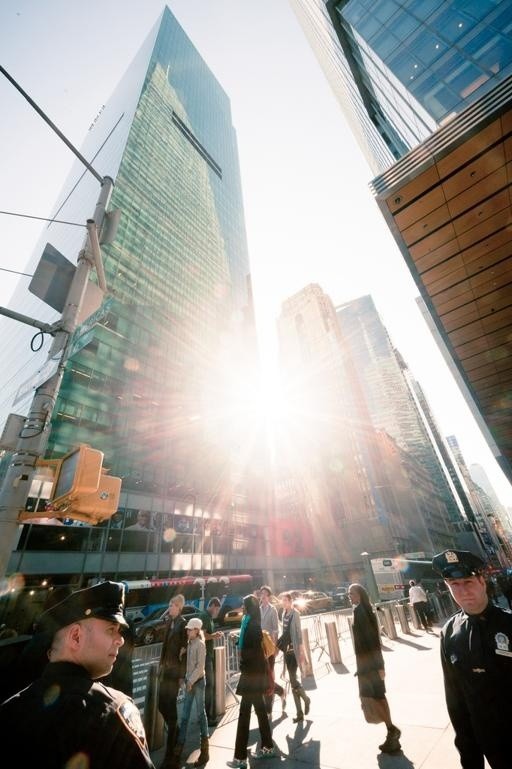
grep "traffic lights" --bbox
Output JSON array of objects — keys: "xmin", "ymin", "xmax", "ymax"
[{"xmin": 46, "ymin": 443, "xmax": 103, "ymax": 505}]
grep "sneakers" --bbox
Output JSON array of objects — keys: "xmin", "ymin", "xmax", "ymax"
[
  {"xmin": 262, "ymin": 746, "xmax": 275, "ymax": 756},
  {"xmin": 227, "ymin": 759, "xmax": 247, "ymax": 769}
]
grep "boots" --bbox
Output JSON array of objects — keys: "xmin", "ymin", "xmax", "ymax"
[
  {"xmin": 287, "ymin": 689, "xmax": 304, "ymax": 722},
  {"xmin": 194, "ymin": 736, "xmax": 209, "ymax": 765},
  {"xmin": 160, "ymin": 744, "xmax": 183, "ymax": 769}
]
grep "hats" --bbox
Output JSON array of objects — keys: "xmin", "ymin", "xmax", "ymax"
[
  {"xmin": 170, "ymin": 594, "xmax": 185, "ymax": 611},
  {"xmin": 31, "ymin": 580, "xmax": 130, "ymax": 635},
  {"xmin": 429, "ymin": 549, "xmax": 485, "ymax": 581},
  {"xmin": 184, "ymin": 618, "xmax": 203, "ymax": 631}
]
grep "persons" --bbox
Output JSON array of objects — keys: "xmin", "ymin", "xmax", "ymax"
[
  {"xmin": 258, "ymin": 586, "xmax": 286, "ymax": 724},
  {"xmin": 43, "ymin": 573, "xmax": 72, "ymax": 613},
  {"xmin": 430, "ymin": 550, "xmax": 512, "ymax": 769},
  {"xmin": 92, "ymin": 581, "xmax": 136, "ymax": 701},
  {"xmin": 198, "ymin": 597, "xmax": 225, "ymax": 727},
  {"xmin": 409, "ymin": 580, "xmax": 433, "ymax": 633},
  {"xmin": 109, "ymin": 508, "xmax": 217, "ymax": 537},
  {"xmin": 157, "ymin": 594, "xmax": 189, "ymax": 769},
  {"xmin": 348, "ymin": 583, "xmax": 401, "ymax": 753},
  {"xmin": 280, "ymin": 593, "xmax": 310, "ymax": 722},
  {"xmin": 226, "ymin": 593, "xmax": 276, "ymax": 769},
  {"xmin": 486, "ymin": 570, "xmax": 512, "ymax": 612},
  {"xmin": 0, "ymin": 581, "xmax": 157, "ymax": 769},
  {"xmin": 0, "ymin": 572, "xmax": 34, "ymax": 639},
  {"xmin": 167, "ymin": 617, "xmax": 210, "ymax": 769}
]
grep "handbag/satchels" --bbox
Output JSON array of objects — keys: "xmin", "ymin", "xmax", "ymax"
[
  {"xmin": 275, "ymin": 613, "xmax": 295, "ymax": 652},
  {"xmin": 260, "ymin": 630, "xmax": 275, "ymax": 658}
]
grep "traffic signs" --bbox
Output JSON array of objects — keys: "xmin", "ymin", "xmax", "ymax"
[
  {"xmin": 67, "ymin": 330, "xmax": 92, "ymax": 358},
  {"xmin": 12, "ymin": 349, "xmax": 64, "ymax": 408},
  {"xmin": 71, "ymin": 299, "xmax": 112, "ymax": 342}
]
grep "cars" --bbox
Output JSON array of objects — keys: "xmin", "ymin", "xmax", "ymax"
[
  {"xmin": 133, "ymin": 605, "xmax": 214, "ymax": 647},
  {"xmin": 278, "ymin": 589, "xmax": 302, "ymax": 603},
  {"xmin": 331, "ymin": 586, "xmax": 354, "ymax": 610},
  {"xmin": 225, "ymin": 594, "xmax": 286, "ymax": 626},
  {"xmin": 293, "ymin": 590, "xmax": 337, "ymax": 617}
]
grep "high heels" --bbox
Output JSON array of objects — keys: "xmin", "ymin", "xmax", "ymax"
[{"xmin": 298, "ymin": 687, "xmax": 311, "ymax": 715}]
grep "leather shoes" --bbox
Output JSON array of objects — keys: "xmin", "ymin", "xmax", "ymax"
[{"xmin": 379, "ymin": 725, "xmax": 401, "ymax": 752}]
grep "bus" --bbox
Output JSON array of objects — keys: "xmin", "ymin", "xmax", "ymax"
[
  {"xmin": 97, "ymin": 575, "xmax": 259, "ymax": 634},
  {"xmin": 371, "ymin": 557, "xmax": 448, "ymax": 602}
]
